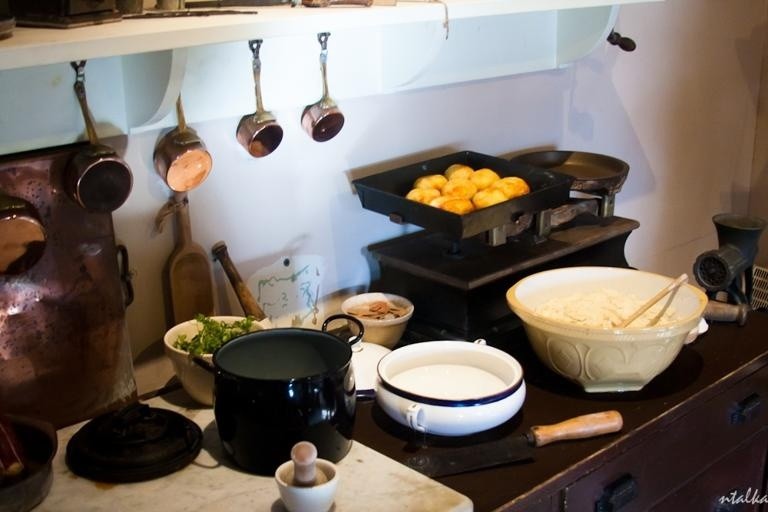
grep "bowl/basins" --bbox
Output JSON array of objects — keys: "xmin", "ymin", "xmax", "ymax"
[
  {"xmin": 274, "ymin": 457, "xmax": 341, "ymax": 511},
  {"xmin": 162, "ymin": 315, "xmax": 266, "ymax": 407},
  {"xmin": 376, "ymin": 338, "xmax": 527, "ymax": 438},
  {"xmin": 504, "ymin": 265, "xmax": 710, "ymax": 394},
  {"xmin": 341, "ymin": 291, "xmax": 416, "ymax": 349}
]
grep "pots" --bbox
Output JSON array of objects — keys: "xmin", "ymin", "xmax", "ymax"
[
  {"xmin": 1, "ymin": 195, "xmax": 49, "ymax": 281},
  {"xmin": 1, "ymin": 413, "xmax": 59, "ymax": 511},
  {"xmin": 64, "ymin": 80, "xmax": 134, "ymax": 215},
  {"xmin": 235, "ymin": 38, "xmax": 283, "ymax": 160},
  {"xmin": 189, "ymin": 314, "xmax": 364, "ymax": 473},
  {"xmin": 151, "ymin": 93, "xmax": 213, "ymax": 194},
  {"xmin": 300, "ymin": 31, "xmax": 346, "ymax": 143}
]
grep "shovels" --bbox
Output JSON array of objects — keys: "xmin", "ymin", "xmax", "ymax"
[{"xmin": 166, "ymin": 189, "xmax": 217, "ymax": 326}]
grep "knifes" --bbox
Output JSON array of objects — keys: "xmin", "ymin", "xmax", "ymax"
[{"xmin": 402, "ymin": 410, "xmax": 624, "ymax": 479}]
